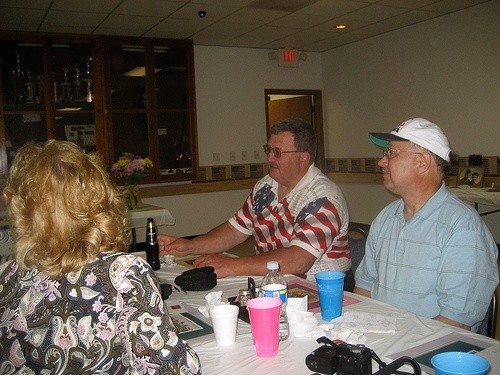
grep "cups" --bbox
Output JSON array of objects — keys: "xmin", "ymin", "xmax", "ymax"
[
  {"xmin": 314, "ymin": 271, "xmax": 346, "ymax": 322},
  {"xmin": 430, "ymin": 351, "xmax": 491, "ymax": 375},
  {"xmin": 209, "ymin": 304, "xmax": 240, "ymax": 350},
  {"xmin": 246, "ymin": 297, "xmax": 282, "ymax": 358}
]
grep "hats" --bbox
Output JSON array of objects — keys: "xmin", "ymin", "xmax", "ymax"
[{"xmin": 368, "ymin": 117, "xmax": 450, "ymax": 163}]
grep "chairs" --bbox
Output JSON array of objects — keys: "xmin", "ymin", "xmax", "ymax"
[{"xmin": 348, "ymin": 221, "xmax": 368, "ymax": 273}]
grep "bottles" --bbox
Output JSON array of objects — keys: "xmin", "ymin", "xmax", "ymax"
[
  {"xmin": 145, "ymin": 217, "xmax": 161, "ymax": 271},
  {"xmin": 239, "ymin": 288, "xmax": 252, "ymax": 307},
  {"xmin": 259, "ymin": 261, "xmax": 289, "ymax": 341},
  {"xmin": 254, "ymin": 286, "xmax": 262, "ymax": 298}
]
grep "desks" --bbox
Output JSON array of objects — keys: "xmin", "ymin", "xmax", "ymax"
[
  {"xmin": 446, "ymin": 185, "xmax": 500, "ymax": 218},
  {"xmin": 130, "ymin": 241, "xmax": 500, "ymax": 375}
]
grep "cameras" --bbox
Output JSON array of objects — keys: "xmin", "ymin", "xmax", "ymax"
[{"xmin": 306, "ymin": 341, "xmax": 372, "ymax": 375}]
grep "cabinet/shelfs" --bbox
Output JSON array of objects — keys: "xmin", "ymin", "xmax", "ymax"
[{"xmin": 0, "ymin": 29, "xmax": 200, "ymax": 184}]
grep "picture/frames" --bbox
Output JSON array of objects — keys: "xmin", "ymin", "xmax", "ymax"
[{"xmin": 456, "ymin": 164, "xmax": 485, "ymax": 188}]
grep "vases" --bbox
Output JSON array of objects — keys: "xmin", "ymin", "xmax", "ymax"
[{"xmin": 123, "ymin": 186, "xmax": 139, "ymax": 211}]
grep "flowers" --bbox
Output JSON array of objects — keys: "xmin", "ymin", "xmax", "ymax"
[{"xmin": 110, "ymin": 152, "xmax": 155, "ymax": 193}]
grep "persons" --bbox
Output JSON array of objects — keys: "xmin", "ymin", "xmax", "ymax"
[
  {"xmin": 350, "ymin": 117, "xmax": 500, "ymax": 333},
  {"xmin": 159, "ymin": 118, "xmax": 356, "ymax": 294},
  {"xmin": 469, "ymin": 173, "xmax": 479, "ymax": 185},
  {"xmin": 0, "ymin": 139, "xmax": 201, "ymax": 375},
  {"xmin": 459, "ymin": 169, "xmax": 471, "ymax": 184}
]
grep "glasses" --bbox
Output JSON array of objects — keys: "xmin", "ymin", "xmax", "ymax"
[
  {"xmin": 263, "ymin": 144, "xmax": 305, "ymax": 157},
  {"xmin": 383, "ymin": 148, "xmax": 422, "ymax": 158}
]
